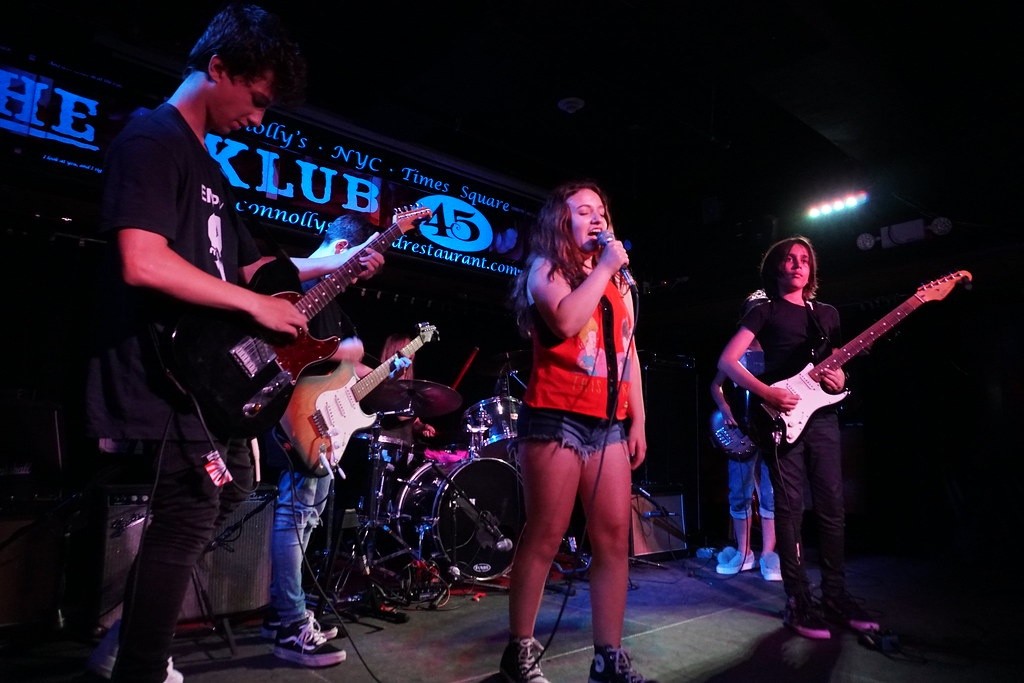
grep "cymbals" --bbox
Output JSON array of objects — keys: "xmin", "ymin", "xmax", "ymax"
[{"xmin": 368, "ymin": 379, "xmax": 461, "ymax": 416}]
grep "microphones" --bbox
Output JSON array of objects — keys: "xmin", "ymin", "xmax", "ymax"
[
  {"xmin": 643, "ymin": 511, "xmax": 678, "ymax": 519},
  {"xmin": 495, "ymin": 363, "xmax": 510, "ymax": 395},
  {"xmin": 596, "ymin": 231, "xmax": 639, "ymax": 295},
  {"xmin": 496, "ymin": 538, "xmax": 513, "ymax": 553}
]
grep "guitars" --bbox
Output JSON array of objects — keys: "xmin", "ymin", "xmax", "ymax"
[
  {"xmin": 706, "ymin": 315, "xmax": 900, "ymax": 461},
  {"xmin": 745, "ymin": 270, "xmax": 973, "ymax": 454},
  {"xmin": 272, "ymin": 321, "xmax": 439, "ymax": 477},
  {"xmin": 155, "ymin": 203, "xmax": 432, "ymax": 440}
]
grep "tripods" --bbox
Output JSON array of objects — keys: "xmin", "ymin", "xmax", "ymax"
[
  {"xmin": 409, "ymin": 463, "xmax": 512, "ymax": 610},
  {"xmin": 323, "ymin": 407, "xmax": 439, "ymax": 612}
]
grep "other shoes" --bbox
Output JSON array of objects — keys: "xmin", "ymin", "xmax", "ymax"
[
  {"xmin": 103, "ymin": 655, "xmax": 184, "ymax": 683},
  {"xmin": 784, "ymin": 601, "xmax": 831, "ymax": 639},
  {"xmin": 847, "ymin": 603, "xmax": 880, "ymax": 631}
]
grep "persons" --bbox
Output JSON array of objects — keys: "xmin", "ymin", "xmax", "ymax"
[
  {"xmin": 83, "ymin": 0, "xmax": 385, "ymax": 683},
  {"xmin": 711, "ymin": 337, "xmax": 782, "ymax": 581},
  {"xmin": 246, "ymin": 215, "xmax": 412, "ymax": 668},
  {"xmin": 717, "ymin": 238, "xmax": 881, "ymax": 638},
  {"xmin": 377, "ymin": 336, "xmax": 436, "ymax": 472},
  {"xmin": 499, "ymin": 182, "xmax": 657, "ymax": 683}
]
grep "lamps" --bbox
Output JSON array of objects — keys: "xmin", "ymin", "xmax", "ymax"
[{"xmin": 856, "ymin": 217, "xmax": 953, "ymax": 249}]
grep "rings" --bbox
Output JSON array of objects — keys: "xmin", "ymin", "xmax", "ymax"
[{"xmin": 833, "ymin": 388, "xmax": 838, "ymax": 391}]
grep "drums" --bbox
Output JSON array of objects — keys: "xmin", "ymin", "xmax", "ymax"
[
  {"xmin": 352, "ymin": 434, "xmax": 414, "ymax": 514},
  {"xmin": 396, "ymin": 456, "xmax": 528, "ymax": 581},
  {"xmin": 464, "ymin": 396, "xmax": 522, "ymax": 457}
]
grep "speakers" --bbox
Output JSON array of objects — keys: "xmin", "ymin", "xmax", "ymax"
[
  {"xmin": 631, "ymin": 363, "xmax": 701, "ymax": 548},
  {"xmin": 86, "ymin": 479, "xmax": 274, "ymax": 633},
  {"xmin": 628, "ymin": 492, "xmax": 689, "ymax": 563},
  {"xmin": 0, "ymin": 497, "xmax": 72, "ymax": 631}
]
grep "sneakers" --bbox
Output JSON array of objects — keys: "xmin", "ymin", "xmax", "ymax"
[
  {"xmin": 262, "ymin": 607, "xmax": 338, "ymax": 643},
  {"xmin": 589, "ymin": 644, "xmax": 658, "ymax": 683},
  {"xmin": 273, "ymin": 617, "xmax": 346, "ymax": 666},
  {"xmin": 500, "ymin": 637, "xmax": 549, "ymax": 683},
  {"xmin": 759, "ymin": 552, "xmax": 783, "ymax": 581},
  {"xmin": 716, "ymin": 550, "xmax": 756, "ymax": 574}
]
